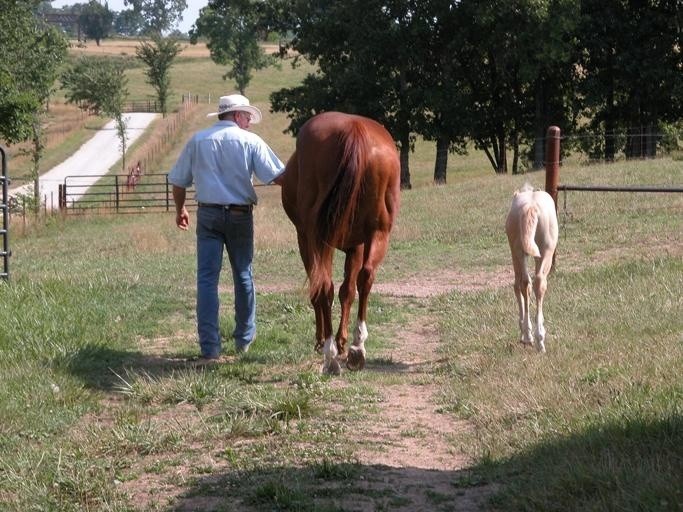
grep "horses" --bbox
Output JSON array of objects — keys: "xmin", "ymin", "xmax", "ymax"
[
  {"xmin": 505, "ymin": 182, "xmax": 559, "ymax": 354},
  {"xmin": 281, "ymin": 111, "xmax": 401, "ymax": 377}
]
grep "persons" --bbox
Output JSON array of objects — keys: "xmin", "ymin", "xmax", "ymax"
[{"xmin": 167, "ymin": 94, "xmax": 288, "ymax": 361}]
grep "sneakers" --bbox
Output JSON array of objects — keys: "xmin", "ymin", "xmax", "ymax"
[{"xmin": 234, "ymin": 329, "xmax": 256, "ymax": 353}]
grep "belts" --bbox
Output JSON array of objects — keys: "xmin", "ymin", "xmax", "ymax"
[{"xmin": 197, "ymin": 202, "xmax": 248, "ymax": 211}]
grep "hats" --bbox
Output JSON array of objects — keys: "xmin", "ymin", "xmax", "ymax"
[{"xmin": 206, "ymin": 94, "xmax": 261, "ymax": 124}]
